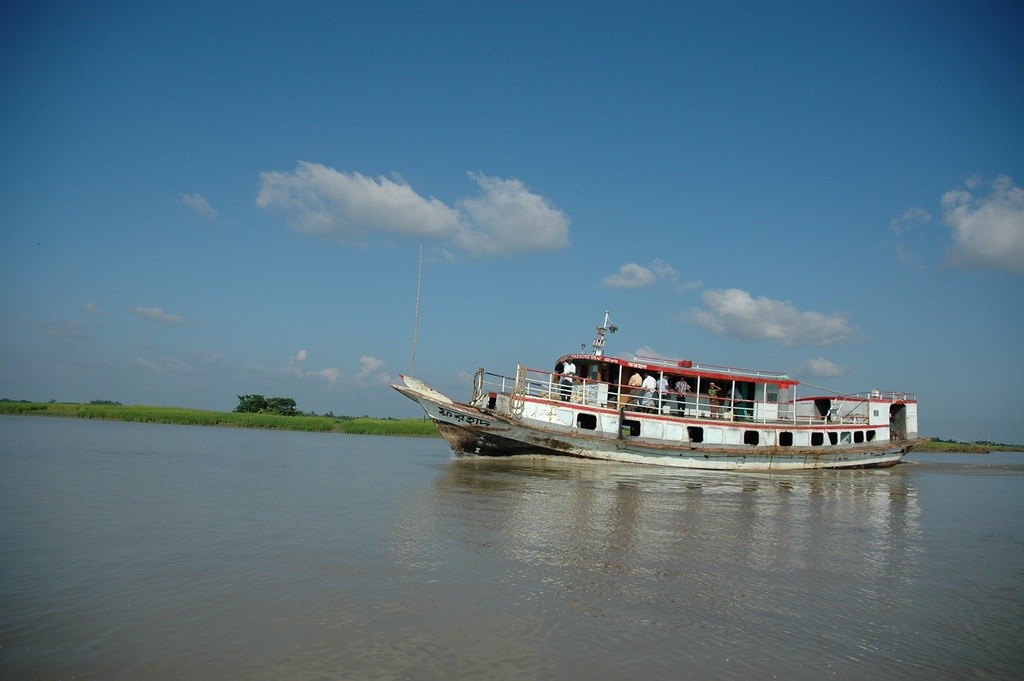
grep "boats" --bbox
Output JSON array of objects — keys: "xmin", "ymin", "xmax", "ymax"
[{"xmin": 388, "ymin": 243, "xmax": 925, "ymax": 471}]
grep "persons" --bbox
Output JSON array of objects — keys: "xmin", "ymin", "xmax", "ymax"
[
  {"xmin": 728, "ymin": 386, "xmax": 745, "ymax": 421},
  {"xmin": 707, "ymin": 383, "xmax": 721, "ymax": 420},
  {"xmin": 673, "ymin": 376, "xmax": 693, "ymax": 418},
  {"xmin": 626, "ymin": 370, "xmax": 672, "ymax": 414},
  {"xmin": 560, "ymin": 354, "xmax": 576, "ymax": 401}
]
